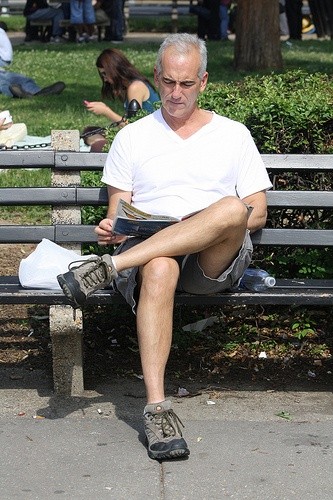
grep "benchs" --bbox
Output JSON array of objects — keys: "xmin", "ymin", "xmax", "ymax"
[
  {"xmin": 26, "ymin": 5, "xmax": 110, "ymax": 43},
  {"xmin": 0, "ymin": 129, "xmax": 333, "ymax": 398}
]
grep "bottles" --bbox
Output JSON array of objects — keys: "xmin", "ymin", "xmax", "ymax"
[{"xmin": 240, "ymin": 269, "xmax": 276, "ymax": 292}]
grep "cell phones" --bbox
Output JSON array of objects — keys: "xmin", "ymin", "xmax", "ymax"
[{"xmin": 84, "ymin": 100, "xmax": 89, "ymax": 104}]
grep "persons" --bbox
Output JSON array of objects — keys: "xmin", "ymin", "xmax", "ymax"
[
  {"xmin": 0, "ymin": 0, "xmax": 128, "ymax": 66},
  {"xmin": 0, "ymin": 69, "xmax": 66, "ymax": 98},
  {"xmin": 187, "ymin": 0, "xmax": 333, "ymax": 45},
  {"xmin": 83, "ymin": 47, "xmax": 161, "ymax": 128},
  {"xmin": 56, "ymin": 33, "xmax": 273, "ymax": 459}
]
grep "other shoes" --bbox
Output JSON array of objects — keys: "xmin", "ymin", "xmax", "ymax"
[
  {"xmin": 48, "ymin": 35, "xmax": 64, "ymax": 43},
  {"xmin": 102, "ymin": 35, "xmax": 123, "ymax": 43},
  {"xmin": 77, "ymin": 33, "xmax": 97, "ymax": 42}
]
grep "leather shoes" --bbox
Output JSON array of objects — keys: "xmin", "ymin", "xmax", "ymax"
[
  {"xmin": 8, "ymin": 83, "xmax": 32, "ymax": 99},
  {"xmin": 32, "ymin": 82, "xmax": 66, "ymax": 96}
]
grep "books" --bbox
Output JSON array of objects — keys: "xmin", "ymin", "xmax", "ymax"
[{"xmin": 110, "ymin": 193, "xmax": 208, "ymax": 245}]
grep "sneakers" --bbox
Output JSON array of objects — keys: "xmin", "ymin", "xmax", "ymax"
[
  {"xmin": 141, "ymin": 400, "xmax": 190, "ymax": 459},
  {"xmin": 56, "ymin": 254, "xmax": 118, "ymax": 310}
]
